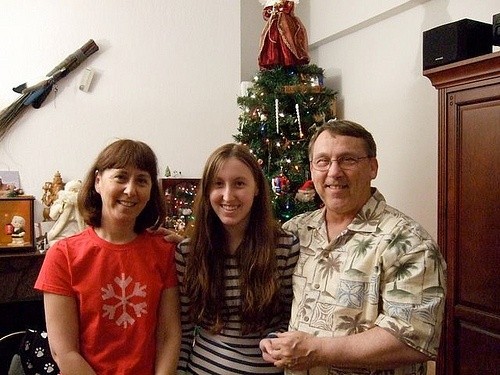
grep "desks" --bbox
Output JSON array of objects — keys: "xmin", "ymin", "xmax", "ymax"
[{"xmin": 0, "ymin": 249, "xmax": 48, "ymax": 303}]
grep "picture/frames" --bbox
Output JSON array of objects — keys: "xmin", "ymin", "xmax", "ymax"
[{"xmin": 0, "ymin": 196, "xmax": 35, "ymax": 255}]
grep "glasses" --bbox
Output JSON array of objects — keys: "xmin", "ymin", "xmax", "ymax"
[{"xmin": 309, "ymin": 155, "xmax": 370, "ymax": 171}]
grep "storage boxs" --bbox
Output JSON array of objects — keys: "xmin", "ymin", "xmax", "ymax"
[{"xmin": 423, "ymin": 18, "xmax": 493, "ymax": 69}]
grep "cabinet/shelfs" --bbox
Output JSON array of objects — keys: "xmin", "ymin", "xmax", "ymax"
[{"xmin": 421, "ymin": 51, "xmax": 500, "ymax": 375}]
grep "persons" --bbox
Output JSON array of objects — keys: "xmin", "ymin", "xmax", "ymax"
[
  {"xmin": 33, "ymin": 139, "xmax": 182, "ymax": 375},
  {"xmin": 10, "ymin": 216, "xmax": 26, "ymax": 244},
  {"xmin": 157, "ymin": 119, "xmax": 447, "ymax": 375},
  {"xmin": 174, "ymin": 143, "xmax": 300, "ymax": 375}
]
